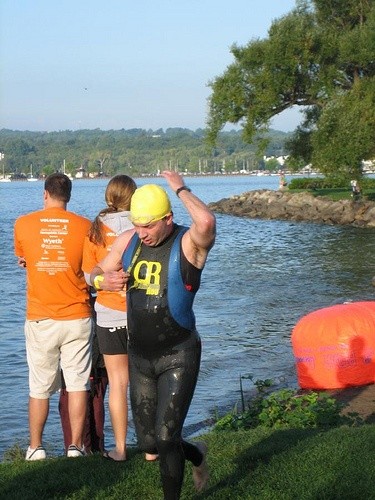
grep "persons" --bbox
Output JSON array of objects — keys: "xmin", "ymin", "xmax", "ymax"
[
  {"xmin": 88, "ymin": 172, "xmax": 216, "ymax": 500},
  {"xmin": 59, "ymin": 275, "xmax": 107, "ymax": 454},
  {"xmin": 82, "ymin": 175, "xmax": 159, "ymax": 462},
  {"xmin": 13, "ymin": 174, "xmax": 96, "ymax": 461}
]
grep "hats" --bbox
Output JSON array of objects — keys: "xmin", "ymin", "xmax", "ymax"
[{"xmin": 130, "ymin": 184, "xmax": 172, "ymax": 223}]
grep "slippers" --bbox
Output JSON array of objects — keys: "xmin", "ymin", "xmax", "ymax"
[{"xmin": 102, "ymin": 451, "xmax": 127, "ymax": 462}]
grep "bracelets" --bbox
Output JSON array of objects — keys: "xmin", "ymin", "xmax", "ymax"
[
  {"xmin": 176, "ymin": 186, "xmax": 191, "ymax": 198},
  {"xmin": 93, "ymin": 275, "xmax": 104, "ymax": 289}
]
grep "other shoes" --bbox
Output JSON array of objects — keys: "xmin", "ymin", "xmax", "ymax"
[
  {"xmin": 24, "ymin": 444, "xmax": 46, "ymax": 461},
  {"xmin": 81, "ymin": 443, "xmax": 101, "ymax": 456},
  {"xmin": 66, "ymin": 444, "xmax": 86, "ymax": 458}
]
